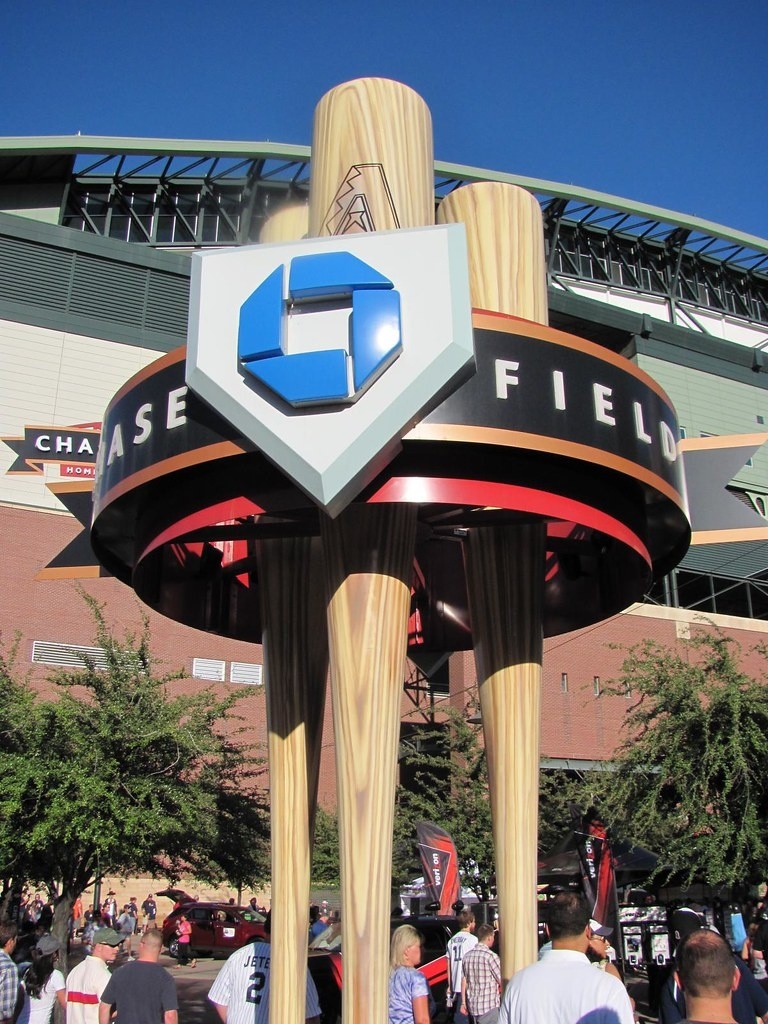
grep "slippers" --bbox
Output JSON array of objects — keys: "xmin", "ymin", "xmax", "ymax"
[
  {"xmin": 174, "ymin": 966, "xmax": 182, "ymax": 969},
  {"xmin": 191, "ymin": 961, "xmax": 196, "ymax": 967}
]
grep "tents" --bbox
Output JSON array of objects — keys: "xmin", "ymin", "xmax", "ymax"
[
  {"xmin": 537, "ymin": 832, "xmax": 711, "ymax": 900},
  {"xmin": 399, "ymin": 858, "xmax": 497, "ymax": 916}
]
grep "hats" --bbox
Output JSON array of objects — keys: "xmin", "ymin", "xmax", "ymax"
[
  {"xmin": 668, "ymin": 910, "xmax": 701, "ymax": 941},
  {"xmin": 35, "ymin": 936, "xmax": 60, "ymax": 955},
  {"xmin": 107, "ymin": 891, "xmax": 116, "ymax": 895},
  {"xmin": 250, "ymin": 898, "xmax": 256, "ymax": 903},
  {"xmin": 93, "ymin": 928, "xmax": 127, "ymax": 945},
  {"xmin": 322, "ymin": 901, "xmax": 327, "ymax": 904},
  {"xmin": 149, "ymin": 894, "xmax": 152, "ymax": 898},
  {"xmin": 589, "ymin": 919, "xmax": 613, "ymax": 936}
]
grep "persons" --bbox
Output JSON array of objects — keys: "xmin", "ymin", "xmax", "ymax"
[
  {"xmin": 129, "ymin": 897, "xmax": 139, "ymax": 934},
  {"xmin": 263, "ymin": 909, "xmax": 271, "ymax": 939},
  {"xmin": 85, "ymin": 904, "xmax": 94, "ymax": 921},
  {"xmin": 195, "ymin": 895, "xmax": 199, "ymax": 901},
  {"xmin": 389, "ymin": 923, "xmax": 436, "ymax": 1024},
  {"xmin": 308, "ymin": 900, "xmax": 341, "ymax": 945},
  {"xmin": 20, "ymin": 890, "xmax": 44, "ymax": 922},
  {"xmin": 141, "ymin": 894, "xmax": 157, "ymax": 933},
  {"xmin": 249, "ymin": 898, "xmax": 265, "ymax": 912},
  {"xmin": 103, "ymin": 890, "xmax": 117, "ymax": 925},
  {"xmin": 173, "ymin": 914, "xmax": 198, "ymax": 968},
  {"xmin": 208, "ymin": 908, "xmax": 322, "ymax": 1024},
  {"xmin": 658, "ymin": 901, "xmax": 768, "ymax": 1024},
  {"xmin": 499, "ymin": 890, "xmax": 639, "ymax": 1024},
  {"xmin": 73, "ymin": 894, "xmax": 83, "ymax": 938},
  {"xmin": 0, "ymin": 903, "xmax": 180, "ymax": 1024},
  {"xmin": 219, "ymin": 897, "xmax": 236, "ymax": 922},
  {"xmin": 446, "ymin": 911, "xmax": 502, "ymax": 1024}
]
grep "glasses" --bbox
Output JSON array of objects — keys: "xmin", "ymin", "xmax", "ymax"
[
  {"xmin": 98, "ymin": 940, "xmax": 124, "ymax": 948},
  {"xmin": 590, "ymin": 936, "xmax": 606, "ymax": 943}
]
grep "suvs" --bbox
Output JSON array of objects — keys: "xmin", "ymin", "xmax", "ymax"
[
  {"xmin": 307, "ymin": 913, "xmax": 462, "ymax": 1024},
  {"xmin": 155, "ymin": 890, "xmax": 268, "ymax": 959}
]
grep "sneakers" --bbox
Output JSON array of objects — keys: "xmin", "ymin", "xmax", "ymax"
[{"xmin": 128, "ymin": 957, "xmax": 135, "ymax": 961}]
